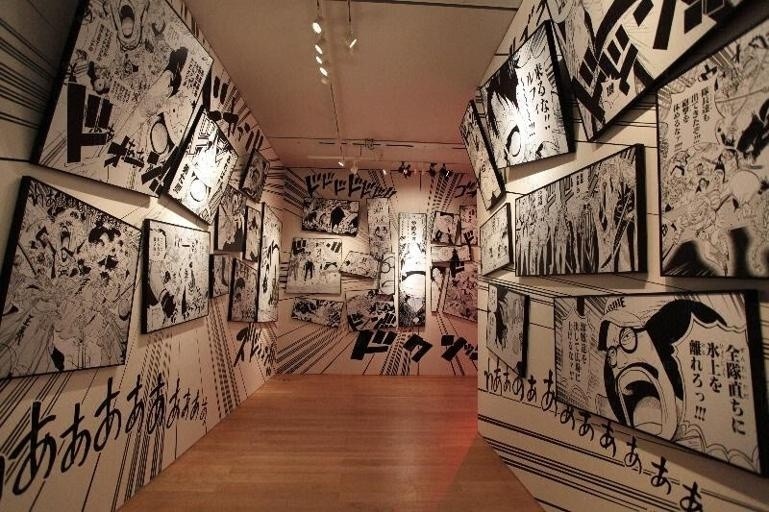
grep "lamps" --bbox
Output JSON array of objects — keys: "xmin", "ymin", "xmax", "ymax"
[
  {"xmin": 319, "ymin": 139, "xmax": 453, "ymax": 179},
  {"xmin": 344, "ymin": 0, "xmax": 360, "ymax": 48},
  {"xmin": 311, "ymin": 0, "xmax": 330, "ymax": 86}
]
